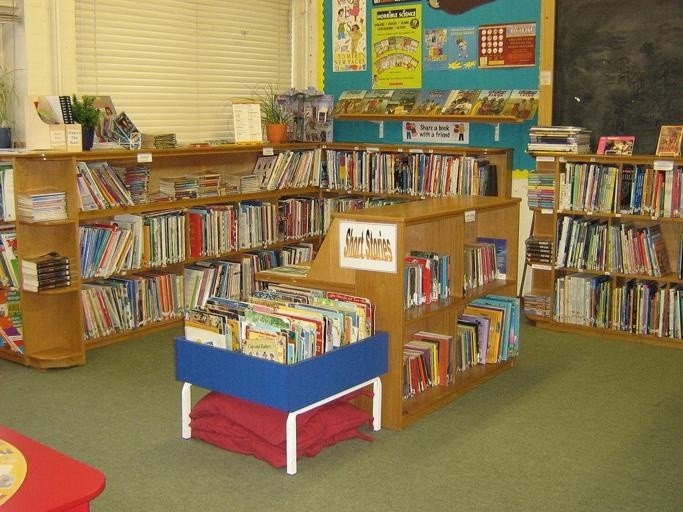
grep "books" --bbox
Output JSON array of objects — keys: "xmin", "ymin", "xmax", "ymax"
[
  {"xmin": 528, "ymin": 125, "xmax": 593, "ymax": 154},
  {"xmin": 597, "ymin": 136, "xmax": 634, "ymax": 156},
  {"xmin": 523, "ymin": 157, "xmax": 683, "ymax": 339},
  {"xmin": 78, "ymin": 148, "xmax": 521, "ymax": 396},
  {"xmin": 279, "ymin": 88, "xmax": 539, "ymax": 143},
  {"xmin": 1, "ymin": 168, "xmax": 71, "ymax": 354},
  {"xmin": 35, "ymin": 94, "xmax": 178, "ymax": 151},
  {"xmin": 656, "ymin": 126, "xmax": 682, "ymax": 157}
]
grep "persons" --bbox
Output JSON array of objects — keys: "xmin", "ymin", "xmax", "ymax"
[
  {"xmin": 347, "ymin": 25, "xmax": 362, "ymax": 57},
  {"xmin": 456, "ymin": 39, "xmax": 468, "ymax": 59},
  {"xmin": 336, "ymin": 9, "xmax": 345, "ymax": 40}
]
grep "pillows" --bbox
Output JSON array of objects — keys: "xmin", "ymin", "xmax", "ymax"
[{"xmin": 189, "ymin": 388, "xmax": 374, "ymax": 469}]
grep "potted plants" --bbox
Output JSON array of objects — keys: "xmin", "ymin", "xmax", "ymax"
[
  {"xmin": 0, "ymin": 64, "xmax": 24, "ymax": 148},
  {"xmin": 71, "ymin": 93, "xmax": 101, "ymax": 150},
  {"xmin": 250, "ymin": 79, "xmax": 295, "ymax": 143}
]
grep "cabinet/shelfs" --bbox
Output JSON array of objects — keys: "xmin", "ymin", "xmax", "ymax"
[{"xmin": 524, "ymin": 150, "xmax": 683, "ymax": 350}]
